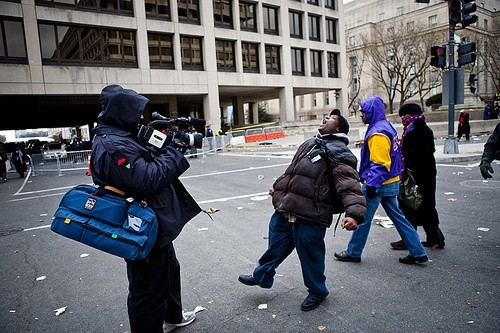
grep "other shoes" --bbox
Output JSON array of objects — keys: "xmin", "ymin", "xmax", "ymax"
[
  {"xmin": 421, "ymin": 240, "xmax": 445, "ymax": 248},
  {"xmin": 391, "ymin": 239, "xmax": 406, "ymax": 249}
]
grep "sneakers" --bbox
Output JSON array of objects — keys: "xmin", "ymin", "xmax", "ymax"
[{"xmin": 163, "ymin": 310, "xmax": 196, "ymax": 333}]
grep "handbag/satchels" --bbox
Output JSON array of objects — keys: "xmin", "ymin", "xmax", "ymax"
[
  {"xmin": 50, "ymin": 184, "xmax": 158, "ymax": 262},
  {"xmin": 399, "ymin": 169, "xmax": 423, "ymax": 209}
]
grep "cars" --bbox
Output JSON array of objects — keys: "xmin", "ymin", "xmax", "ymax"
[{"xmin": 41, "ymin": 143, "xmax": 67, "ymax": 160}]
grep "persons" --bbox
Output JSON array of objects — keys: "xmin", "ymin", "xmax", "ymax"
[
  {"xmin": 238, "ymin": 107, "xmax": 367, "ymax": 310},
  {"xmin": 479, "ymin": 123, "xmax": 500, "ymax": 179},
  {"xmin": 11, "ymin": 144, "xmax": 27, "ymax": 178},
  {"xmin": 0, "ymin": 143, "xmax": 8, "ymax": 182},
  {"xmin": 483, "ymin": 101, "xmax": 500, "ymax": 120},
  {"xmin": 457, "ymin": 110, "xmax": 470, "ymax": 141},
  {"xmin": 189, "ymin": 128, "xmax": 197, "ymax": 158},
  {"xmin": 390, "ymin": 103, "xmax": 446, "ymax": 250},
  {"xmin": 335, "ymin": 97, "xmax": 428, "ymax": 264},
  {"xmin": 207, "ymin": 125, "xmax": 214, "ymax": 149},
  {"xmin": 60, "ymin": 137, "xmax": 85, "ymax": 163},
  {"xmin": 90, "ymin": 86, "xmax": 220, "ymax": 333}
]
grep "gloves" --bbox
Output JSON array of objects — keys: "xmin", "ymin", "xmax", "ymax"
[{"xmin": 480, "ymin": 157, "xmax": 494, "ymax": 179}]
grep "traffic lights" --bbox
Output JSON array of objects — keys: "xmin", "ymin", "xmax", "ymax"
[
  {"xmin": 448, "ymin": 0, "xmax": 478, "ymax": 27},
  {"xmin": 430, "ymin": 45, "xmax": 447, "ymax": 68}
]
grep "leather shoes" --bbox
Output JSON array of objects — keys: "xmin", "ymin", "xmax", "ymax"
[
  {"xmin": 334, "ymin": 251, "xmax": 361, "ymax": 263},
  {"xmin": 239, "ymin": 275, "xmax": 258, "ymax": 286},
  {"xmin": 399, "ymin": 255, "xmax": 428, "ymax": 264},
  {"xmin": 301, "ymin": 296, "xmax": 322, "ymax": 311}
]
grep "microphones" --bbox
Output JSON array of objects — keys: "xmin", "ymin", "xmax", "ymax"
[
  {"xmin": 180, "ymin": 117, "xmax": 206, "ymax": 127},
  {"xmin": 151, "ymin": 112, "xmax": 171, "ymax": 121}
]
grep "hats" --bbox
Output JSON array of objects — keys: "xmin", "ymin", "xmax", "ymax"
[
  {"xmin": 330, "ymin": 109, "xmax": 349, "ymax": 133},
  {"xmin": 399, "ymin": 103, "xmax": 423, "ymax": 116}
]
